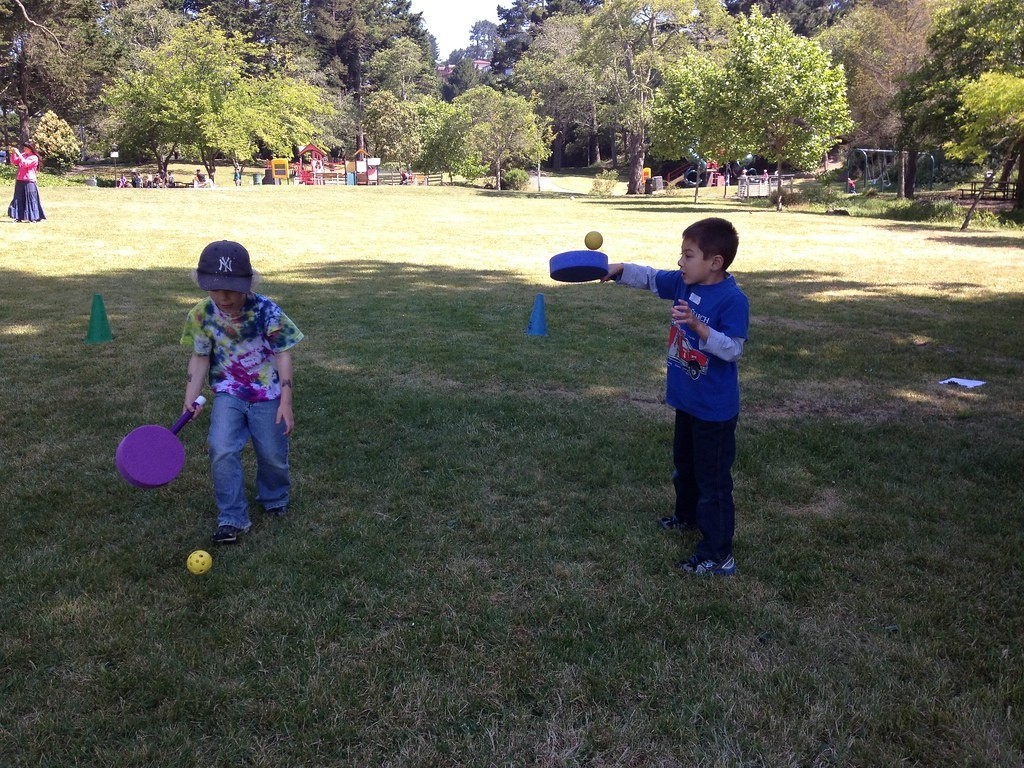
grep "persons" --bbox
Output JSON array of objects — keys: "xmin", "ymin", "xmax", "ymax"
[
  {"xmin": 401, "ymin": 170, "xmax": 409, "ymax": 186},
  {"xmin": 686, "ymin": 148, "xmax": 778, "ymax": 187},
  {"xmin": 598, "ymin": 217, "xmax": 749, "ymax": 576},
  {"xmin": 847, "ymin": 178, "xmax": 856, "ymax": 194},
  {"xmin": 120, "ymin": 167, "xmax": 241, "ymax": 189},
  {"xmin": 179, "ymin": 241, "xmax": 304, "ymax": 544},
  {"xmin": 7, "ymin": 141, "xmax": 46, "ymax": 223}
]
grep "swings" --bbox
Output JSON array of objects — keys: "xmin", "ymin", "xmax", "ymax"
[
  {"xmin": 877, "ymin": 152, "xmax": 891, "ymax": 188},
  {"xmin": 866, "ymin": 152, "xmax": 877, "ymax": 186}
]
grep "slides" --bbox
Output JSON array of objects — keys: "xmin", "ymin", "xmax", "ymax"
[
  {"xmin": 732, "ymin": 147, "xmax": 761, "ymax": 180},
  {"xmin": 683, "ymin": 140, "xmax": 707, "ymax": 187}
]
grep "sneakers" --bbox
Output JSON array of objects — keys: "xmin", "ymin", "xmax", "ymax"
[
  {"xmin": 268, "ymin": 506, "xmax": 288, "ymax": 518},
  {"xmin": 673, "ymin": 552, "xmax": 736, "ymax": 577},
  {"xmin": 212, "ymin": 524, "xmax": 249, "ymax": 542}
]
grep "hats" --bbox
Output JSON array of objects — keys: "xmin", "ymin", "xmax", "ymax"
[{"xmin": 196, "ymin": 239, "xmax": 253, "ymax": 294}]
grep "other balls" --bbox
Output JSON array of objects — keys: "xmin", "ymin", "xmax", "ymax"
[
  {"xmin": 584, "ymin": 230, "xmax": 604, "ymax": 250},
  {"xmin": 186, "ymin": 549, "xmax": 214, "ymax": 576}
]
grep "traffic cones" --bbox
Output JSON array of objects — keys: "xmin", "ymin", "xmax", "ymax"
[
  {"xmin": 83, "ymin": 293, "xmax": 113, "ymax": 343},
  {"xmin": 525, "ymin": 293, "xmax": 550, "ymax": 337}
]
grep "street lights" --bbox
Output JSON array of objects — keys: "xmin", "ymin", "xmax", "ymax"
[{"xmin": 109, "ymin": 142, "xmax": 119, "ymax": 187}]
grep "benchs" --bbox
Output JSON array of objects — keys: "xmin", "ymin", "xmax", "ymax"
[{"xmin": 955, "ymin": 187, "xmax": 1015, "ymax": 201}]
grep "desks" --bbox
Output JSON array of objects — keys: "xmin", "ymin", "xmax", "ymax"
[{"xmin": 965, "ymin": 180, "xmax": 1018, "ymax": 185}]
